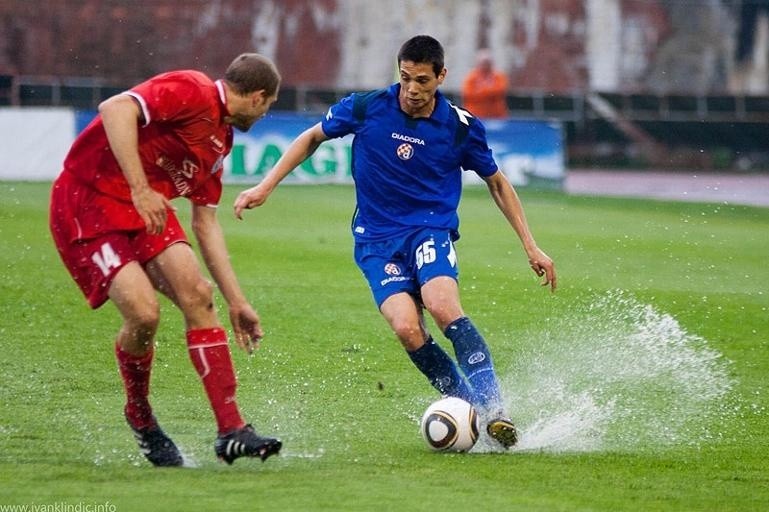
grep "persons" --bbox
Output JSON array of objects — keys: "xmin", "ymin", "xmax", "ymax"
[
  {"xmin": 49, "ymin": 52, "xmax": 282, "ymax": 470},
  {"xmin": 236, "ymin": 36, "xmax": 558, "ymax": 451},
  {"xmin": 462, "ymin": 48, "xmax": 510, "ymax": 117}
]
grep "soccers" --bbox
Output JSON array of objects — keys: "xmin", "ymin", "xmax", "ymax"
[{"xmin": 422, "ymin": 397, "xmax": 480, "ymax": 453}]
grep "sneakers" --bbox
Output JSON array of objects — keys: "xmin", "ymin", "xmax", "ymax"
[
  {"xmin": 213, "ymin": 424, "xmax": 284, "ymax": 466},
  {"xmin": 486, "ymin": 417, "xmax": 519, "ymax": 451},
  {"xmin": 126, "ymin": 416, "xmax": 185, "ymax": 468}
]
